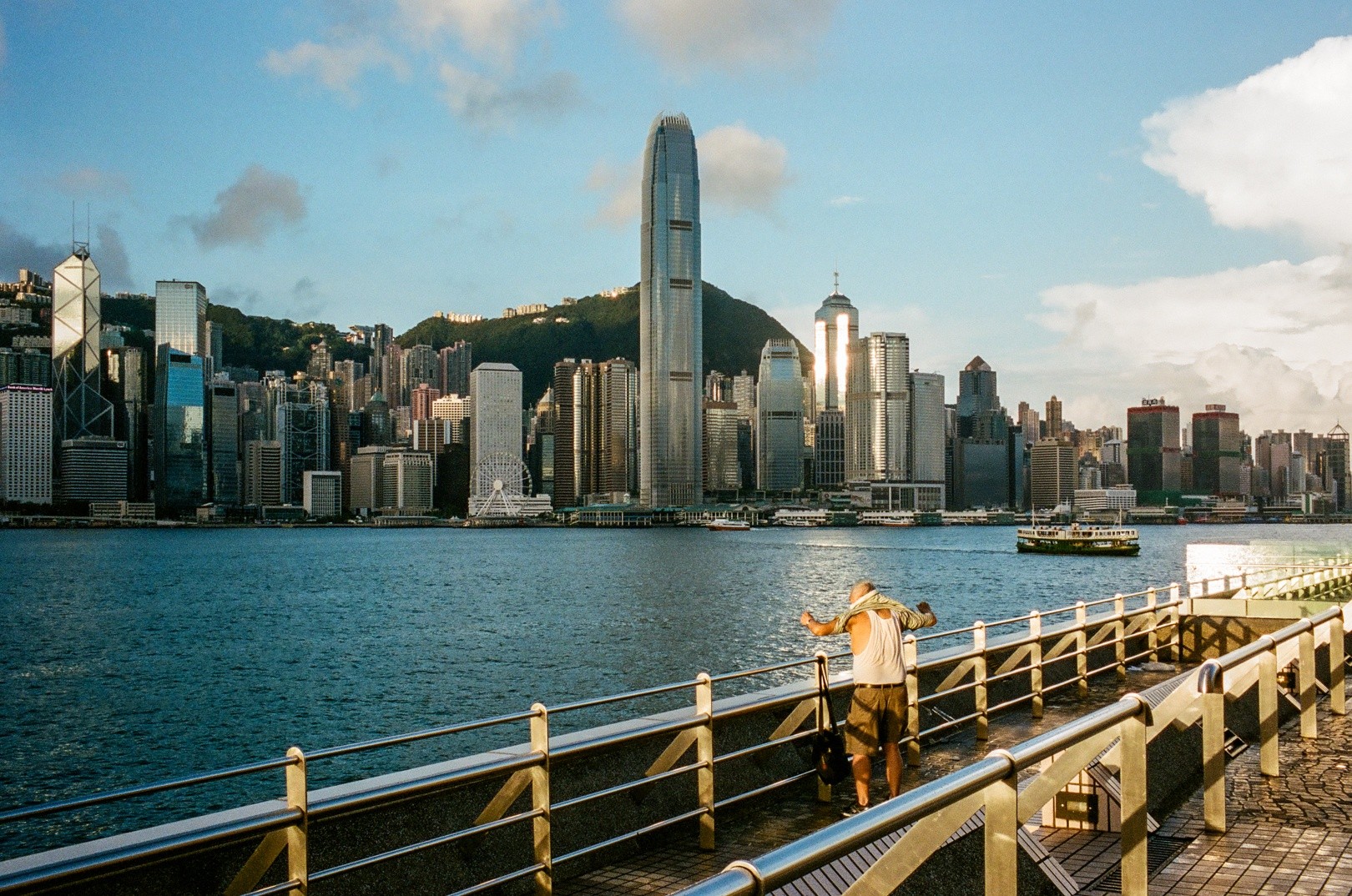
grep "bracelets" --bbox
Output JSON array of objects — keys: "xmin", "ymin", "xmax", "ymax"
[{"xmin": 807, "ymin": 619, "xmax": 812, "ymax": 628}]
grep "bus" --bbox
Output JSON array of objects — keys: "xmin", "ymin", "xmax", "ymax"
[{"xmin": 261, "ymin": 504, "xmax": 304, "ymax": 520}]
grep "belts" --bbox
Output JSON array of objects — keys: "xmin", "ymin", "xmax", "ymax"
[{"xmin": 856, "ymin": 683, "xmax": 905, "ymax": 688}]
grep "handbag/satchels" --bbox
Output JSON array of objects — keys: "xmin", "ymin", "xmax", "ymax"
[{"xmin": 813, "ymin": 728, "xmax": 850, "ymax": 786}]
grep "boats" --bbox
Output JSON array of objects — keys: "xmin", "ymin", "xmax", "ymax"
[
  {"xmin": 703, "ymin": 517, "xmax": 751, "ymax": 530},
  {"xmin": 1012, "ymin": 504, "xmax": 1144, "ymax": 558},
  {"xmin": 882, "ymin": 518, "xmax": 915, "ymax": 527}
]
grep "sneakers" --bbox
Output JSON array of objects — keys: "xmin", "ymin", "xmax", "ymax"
[{"xmin": 841, "ymin": 802, "xmax": 872, "ymax": 816}]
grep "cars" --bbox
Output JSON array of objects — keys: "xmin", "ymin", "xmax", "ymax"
[{"xmin": 290, "ymin": 519, "xmax": 302, "ymax": 524}]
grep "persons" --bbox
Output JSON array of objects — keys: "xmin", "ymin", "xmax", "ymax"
[{"xmin": 800, "ymin": 580, "xmax": 938, "ymax": 818}]
